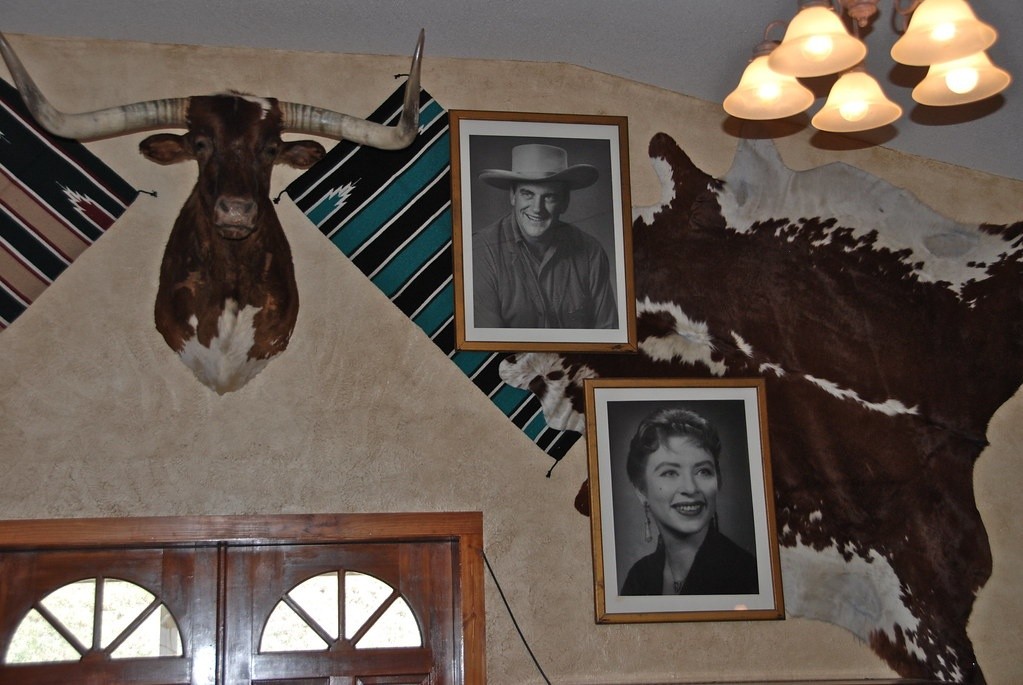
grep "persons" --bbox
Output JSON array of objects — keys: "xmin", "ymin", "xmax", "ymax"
[
  {"xmin": 473, "ymin": 144, "xmax": 620, "ymax": 329},
  {"xmin": 621, "ymin": 411, "xmax": 760, "ymax": 595}
]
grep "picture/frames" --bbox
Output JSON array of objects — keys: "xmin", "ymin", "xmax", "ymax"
[
  {"xmin": 448, "ymin": 108, "xmax": 638, "ymax": 353},
  {"xmin": 583, "ymin": 376, "xmax": 787, "ymax": 623}
]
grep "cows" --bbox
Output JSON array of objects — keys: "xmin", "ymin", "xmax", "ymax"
[{"xmin": 0, "ymin": 28, "xmax": 428, "ymax": 398}]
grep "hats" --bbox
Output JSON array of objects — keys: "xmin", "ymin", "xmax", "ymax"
[{"xmin": 479, "ymin": 144, "xmax": 598, "ymax": 190}]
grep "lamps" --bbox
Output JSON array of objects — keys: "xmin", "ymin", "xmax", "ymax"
[{"xmin": 722, "ymin": 1, "xmax": 1010, "ymax": 133}]
